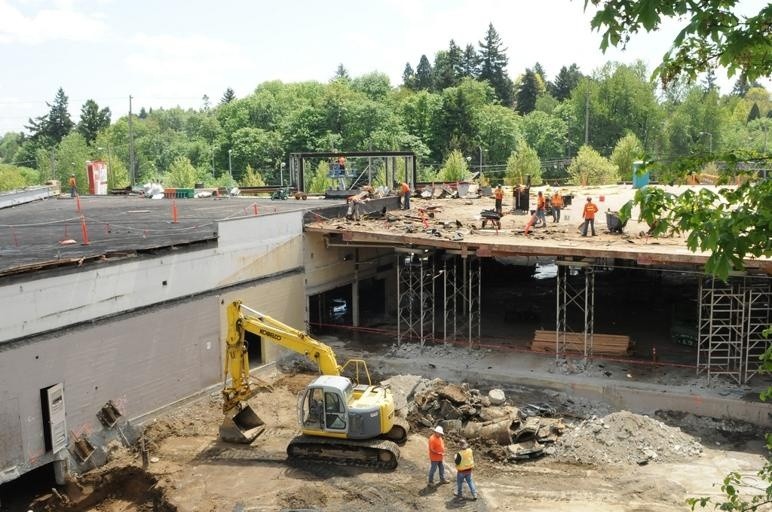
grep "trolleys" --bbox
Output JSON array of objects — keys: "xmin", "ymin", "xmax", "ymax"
[
  {"xmin": 604, "ymin": 208, "xmax": 632, "ymax": 234},
  {"xmin": 480, "ymin": 207, "xmax": 504, "ymax": 230}
]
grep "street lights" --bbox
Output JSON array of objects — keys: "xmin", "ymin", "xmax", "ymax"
[
  {"xmin": 279, "ymin": 162, "xmax": 288, "ymax": 186},
  {"xmin": 465, "ymin": 156, "xmax": 474, "ymax": 170},
  {"xmin": 227, "ymin": 149, "xmax": 232, "ymax": 175},
  {"xmin": 700, "ymin": 131, "xmax": 713, "ymax": 161}
]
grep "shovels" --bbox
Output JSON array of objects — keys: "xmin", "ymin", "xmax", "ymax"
[{"xmin": 577, "ymin": 221, "xmax": 586, "ymax": 233}]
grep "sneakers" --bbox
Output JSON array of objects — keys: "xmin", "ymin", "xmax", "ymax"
[
  {"xmin": 428, "ymin": 482, "xmax": 435, "ymax": 487},
  {"xmin": 441, "ymin": 479, "xmax": 449, "ymax": 484},
  {"xmin": 454, "ymin": 492, "xmax": 462, "ymax": 497},
  {"xmin": 473, "ymin": 495, "xmax": 477, "ymax": 499}
]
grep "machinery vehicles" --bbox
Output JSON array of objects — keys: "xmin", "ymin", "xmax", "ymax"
[{"xmin": 217, "ymin": 299, "xmax": 409, "ymax": 473}]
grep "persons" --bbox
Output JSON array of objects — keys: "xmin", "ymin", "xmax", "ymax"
[
  {"xmin": 493, "ymin": 184, "xmax": 505, "ymax": 217},
  {"xmin": 427, "ymin": 425, "xmax": 450, "ymax": 486},
  {"xmin": 338, "ymin": 155, "xmax": 347, "ymax": 175},
  {"xmin": 453, "ymin": 438, "xmax": 478, "ymax": 499},
  {"xmin": 549, "ymin": 189, "xmax": 564, "ymax": 223},
  {"xmin": 69, "ymin": 173, "xmax": 78, "ymax": 197},
  {"xmin": 532, "ymin": 191, "xmax": 546, "ymax": 228},
  {"xmin": 396, "ymin": 180, "xmax": 411, "ymax": 210},
  {"xmin": 579, "ymin": 196, "xmax": 599, "ymax": 236}
]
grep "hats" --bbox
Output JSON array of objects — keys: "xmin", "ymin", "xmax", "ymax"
[
  {"xmin": 457, "ymin": 440, "xmax": 468, "ymax": 449},
  {"xmin": 434, "ymin": 426, "xmax": 445, "ymax": 435}
]
